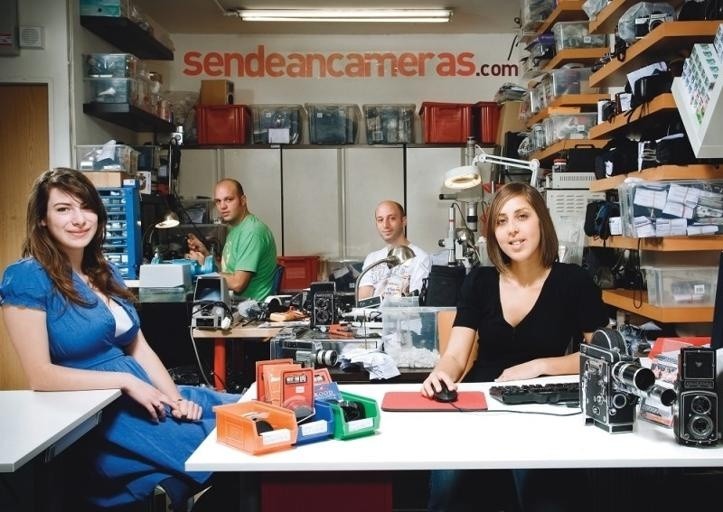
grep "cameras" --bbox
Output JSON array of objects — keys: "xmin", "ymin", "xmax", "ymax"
[
  {"xmin": 284, "ymin": 340, "xmax": 338, "ymax": 371},
  {"xmin": 633, "ymin": 11, "xmax": 673, "ymax": 41},
  {"xmin": 575, "ymin": 326, "xmax": 656, "ymax": 433},
  {"xmin": 661, "ymin": 346, "xmax": 722, "ymax": 448}
]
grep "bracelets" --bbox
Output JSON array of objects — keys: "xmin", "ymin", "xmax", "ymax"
[{"xmin": 173, "ymin": 399, "xmax": 183, "ymax": 403}]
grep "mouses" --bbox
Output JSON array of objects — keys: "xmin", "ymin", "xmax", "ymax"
[{"xmin": 431, "ymin": 380, "xmax": 458, "ymax": 401}]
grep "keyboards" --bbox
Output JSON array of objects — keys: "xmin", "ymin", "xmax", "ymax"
[{"xmin": 489, "ymin": 380, "xmax": 580, "ymax": 406}]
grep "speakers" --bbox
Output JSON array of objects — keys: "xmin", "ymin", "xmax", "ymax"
[{"xmin": 20, "ymin": 26, "xmax": 46, "ymax": 49}]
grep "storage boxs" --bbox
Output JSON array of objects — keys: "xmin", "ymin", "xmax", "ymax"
[
  {"xmin": 619, "ymin": 179, "xmax": 723, "ymax": 239},
  {"xmin": 377, "ymin": 305, "xmax": 457, "ymax": 369},
  {"xmin": 73, "ymin": 144, "xmax": 134, "ymax": 172},
  {"xmin": 135, "ymin": 75, "xmax": 156, "ymax": 115},
  {"xmin": 319, "ymin": 258, "xmax": 363, "ymax": 292},
  {"xmin": 528, "ymin": 114, "xmax": 596, "ymax": 152},
  {"xmin": 130, "ymin": 145, "xmax": 160, "ymax": 171},
  {"xmin": 304, "ymin": 103, "xmax": 362, "ymax": 144},
  {"xmin": 248, "ymin": 105, "xmax": 306, "ymax": 145},
  {"xmin": 640, "ymin": 265, "xmax": 719, "ymax": 307},
  {"xmin": 81, "ymin": 53, "xmax": 139, "ymax": 80},
  {"xmin": 83, "ymin": 79, "xmax": 138, "ymax": 106},
  {"xmin": 277, "ymin": 256, "xmax": 320, "ymax": 289},
  {"xmin": 419, "ymin": 102, "xmax": 476, "ymax": 143},
  {"xmin": 551, "ymin": 20, "xmax": 606, "ymax": 55},
  {"xmin": 362, "ymin": 103, "xmax": 416, "ymax": 145},
  {"xmin": 127, "ymin": 150, "xmax": 142, "ymax": 176},
  {"xmin": 528, "ymin": 67, "xmax": 599, "ymax": 113},
  {"xmin": 472, "ymin": 101, "xmax": 500, "ymax": 144},
  {"xmin": 193, "ymin": 104, "xmax": 254, "ymax": 145}
]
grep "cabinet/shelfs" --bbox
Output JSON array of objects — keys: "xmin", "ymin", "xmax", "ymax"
[
  {"xmin": 526, "ymin": 0, "xmax": 612, "ymax": 169},
  {"xmin": 80, "ymin": 0, "xmax": 175, "ymax": 205},
  {"xmin": 584, "ymin": 0, "xmax": 723, "ymax": 323}
]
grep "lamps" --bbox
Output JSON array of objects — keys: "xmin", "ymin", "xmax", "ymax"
[
  {"xmin": 226, "ymin": 8, "xmax": 454, "ymax": 23},
  {"xmin": 342, "ymin": 245, "xmax": 416, "ymax": 321},
  {"xmin": 443, "ymin": 144, "xmax": 541, "ymax": 189},
  {"xmin": 142, "ymin": 210, "xmax": 180, "ymax": 264}
]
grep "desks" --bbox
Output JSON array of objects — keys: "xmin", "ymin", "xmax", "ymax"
[
  {"xmin": 184, "ymin": 374, "xmax": 723, "ymax": 512},
  {"xmin": 193, "ymin": 320, "xmax": 295, "ymax": 393},
  {"xmin": 0, "ymin": 389, "xmax": 124, "ymax": 473}
]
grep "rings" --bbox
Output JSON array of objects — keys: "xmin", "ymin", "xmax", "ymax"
[{"xmin": 157, "ymin": 403, "xmax": 162, "ymax": 408}]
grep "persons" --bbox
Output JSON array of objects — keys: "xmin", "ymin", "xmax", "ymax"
[
  {"xmin": 358, "ymin": 200, "xmax": 432, "ymax": 301},
  {"xmin": 0, "ymin": 167, "xmax": 244, "ymax": 511},
  {"xmin": 184, "ymin": 178, "xmax": 277, "ymax": 304},
  {"xmin": 420, "ymin": 180, "xmax": 609, "ymax": 511}
]
